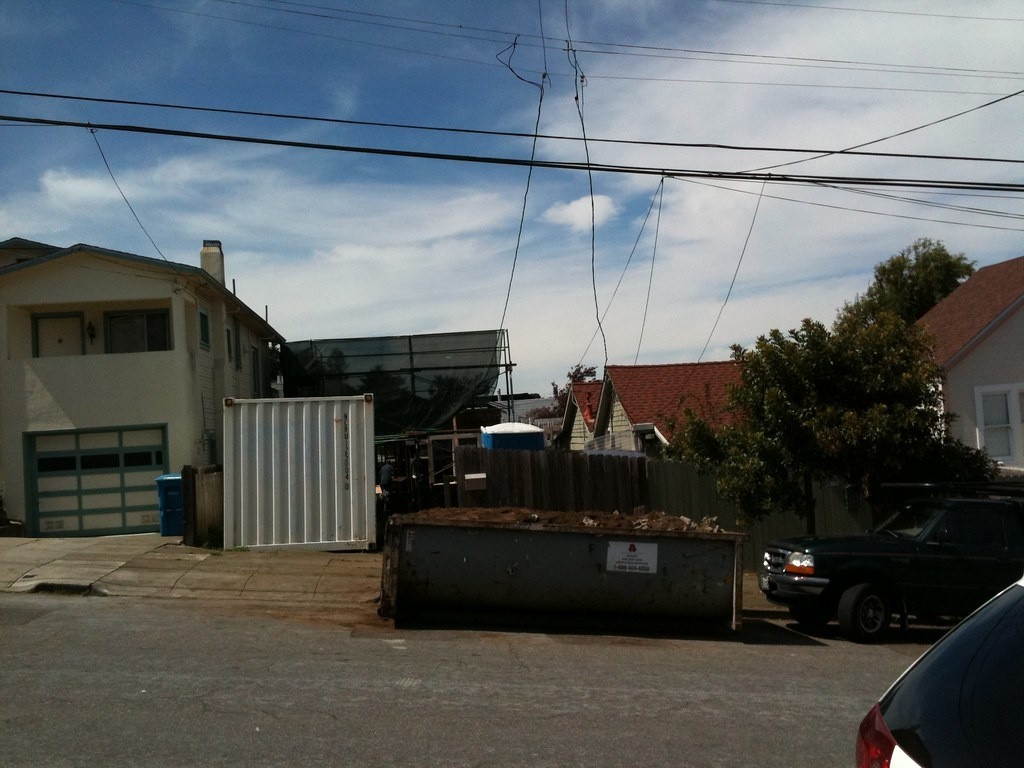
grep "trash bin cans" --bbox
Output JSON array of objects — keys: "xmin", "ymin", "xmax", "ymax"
[{"xmin": 153, "ymin": 473, "xmax": 186, "ymax": 537}]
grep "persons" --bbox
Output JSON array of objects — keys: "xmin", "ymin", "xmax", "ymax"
[{"xmin": 378, "ymin": 460, "xmax": 395, "ymax": 511}]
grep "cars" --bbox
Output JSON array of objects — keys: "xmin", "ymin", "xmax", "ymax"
[{"xmin": 854, "ymin": 576, "xmax": 1024, "ymax": 767}]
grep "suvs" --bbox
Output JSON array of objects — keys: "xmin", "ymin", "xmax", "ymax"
[{"xmin": 759, "ymin": 489, "xmax": 1024, "ymax": 639}]
